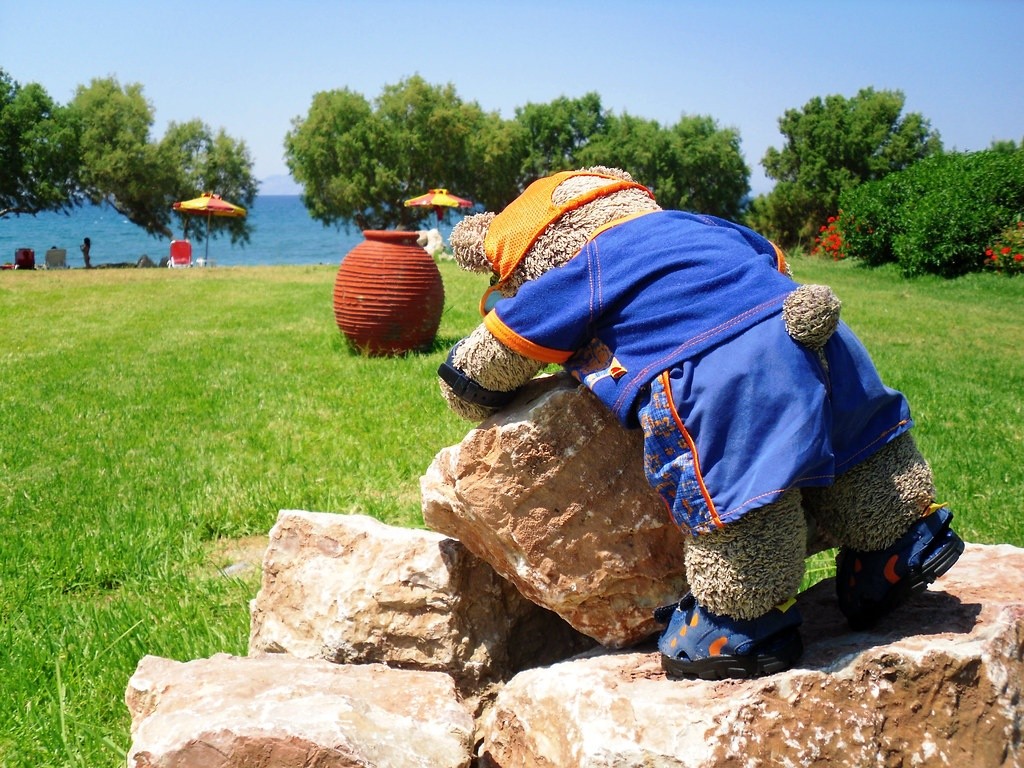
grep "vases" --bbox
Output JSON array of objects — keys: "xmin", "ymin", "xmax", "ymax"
[{"xmin": 335, "ymin": 229, "xmax": 445, "ymax": 353}]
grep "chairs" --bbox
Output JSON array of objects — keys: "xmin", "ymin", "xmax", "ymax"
[
  {"xmin": 42, "ymin": 249, "xmax": 68, "ymax": 270},
  {"xmin": 13, "ymin": 247, "xmax": 38, "ymax": 270},
  {"xmin": 167, "ymin": 240, "xmax": 192, "ymax": 268}
]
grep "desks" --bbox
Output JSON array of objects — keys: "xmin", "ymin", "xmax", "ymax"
[
  {"xmin": 196, "ymin": 257, "xmax": 217, "ymax": 267},
  {"xmin": 0, "ymin": 265, "xmax": 15, "ymax": 270}
]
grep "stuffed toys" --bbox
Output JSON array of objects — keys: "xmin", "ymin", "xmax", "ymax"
[{"xmin": 438, "ymin": 166, "xmax": 965, "ymax": 681}]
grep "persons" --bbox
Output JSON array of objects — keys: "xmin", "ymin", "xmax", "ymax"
[
  {"xmin": 51, "ymin": 246, "xmax": 67, "ymax": 268},
  {"xmin": 80, "ymin": 237, "xmax": 92, "ymax": 268}
]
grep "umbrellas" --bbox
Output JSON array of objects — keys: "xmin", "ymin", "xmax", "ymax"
[
  {"xmin": 173, "ymin": 191, "xmax": 246, "ymax": 267},
  {"xmin": 403, "ymin": 188, "xmax": 473, "ymax": 232}
]
